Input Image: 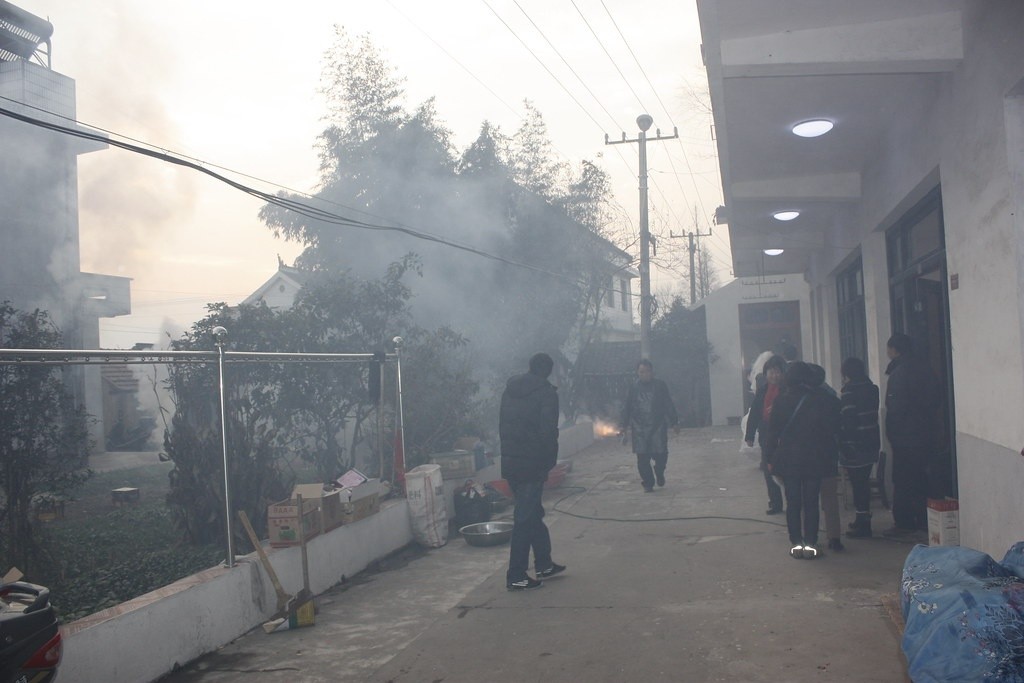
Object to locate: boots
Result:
[845,511,873,538]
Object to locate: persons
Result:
[882,333,929,536]
[839,358,880,540]
[738,342,841,561]
[618,359,680,492]
[499,352,566,592]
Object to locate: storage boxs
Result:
[35,500,63,523]
[431,450,473,478]
[455,437,486,471]
[113,487,139,509]
[295,483,348,534]
[339,470,380,525]
[268,496,316,549]
[927,496,959,547]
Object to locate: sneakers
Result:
[535,562,567,580]
[790,543,822,558]
[882,524,928,542]
[507,568,543,591]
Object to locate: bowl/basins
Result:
[458,522,513,546]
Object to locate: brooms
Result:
[236,505,309,624]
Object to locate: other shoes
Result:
[642,478,655,493]
[653,466,665,486]
[826,537,843,551]
[766,498,783,515]
